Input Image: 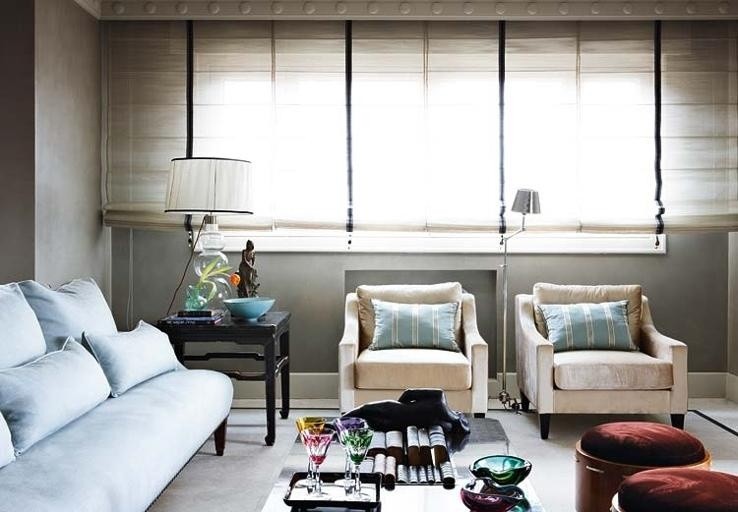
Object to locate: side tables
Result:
[158,312,290,445]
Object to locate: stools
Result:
[572,421,711,512]
[609,467,738,512]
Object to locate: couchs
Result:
[1,277,234,512]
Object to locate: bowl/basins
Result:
[460,477,525,512]
[468,455,532,487]
[223,297,276,322]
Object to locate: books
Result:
[158,309,222,324]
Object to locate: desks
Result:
[261,416,545,512]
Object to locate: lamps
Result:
[499,189,541,409]
[164,157,253,318]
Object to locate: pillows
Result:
[20,279,119,352]
[368,298,461,351]
[0,336,112,456]
[540,300,638,352]
[1,283,47,368]
[82,318,176,398]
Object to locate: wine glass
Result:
[295,416,327,485]
[340,427,373,501]
[332,417,368,486]
[300,428,336,500]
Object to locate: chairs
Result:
[515,283,688,440]
[339,279,489,418]
